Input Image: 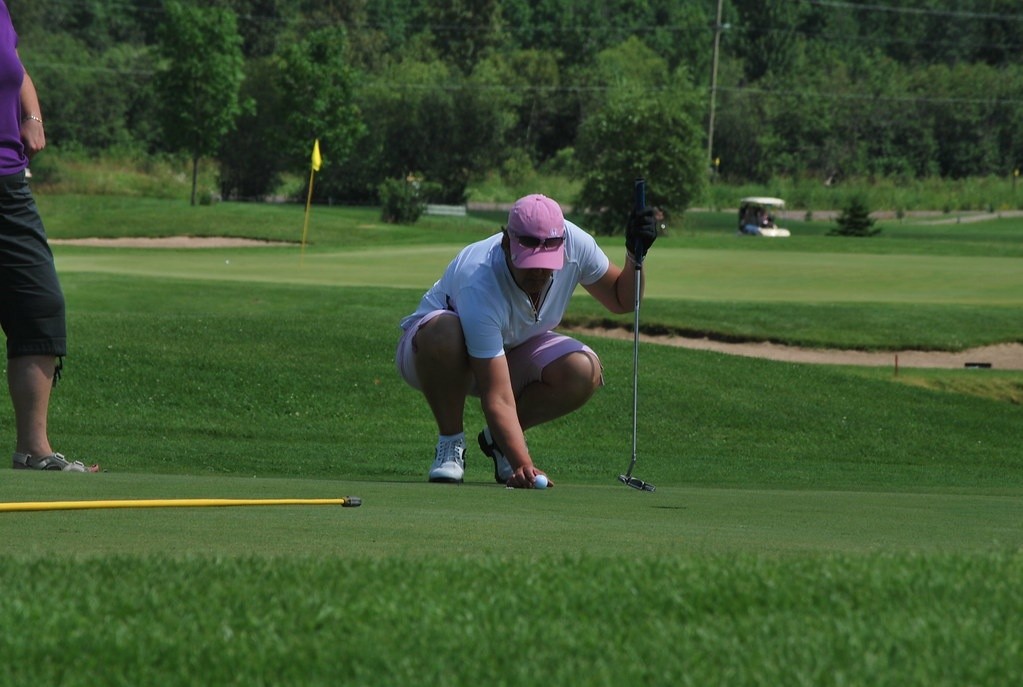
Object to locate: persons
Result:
[0,0,100,473]
[396,193,660,489]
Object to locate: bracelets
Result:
[23,116,42,123]
[626,252,645,263]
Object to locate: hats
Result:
[507,194,565,270]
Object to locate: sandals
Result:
[13,451,98,474]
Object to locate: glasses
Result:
[508,228,566,252]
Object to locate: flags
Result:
[311,139,322,171]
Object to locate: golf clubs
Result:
[0,495,363,513]
[617,176,657,492]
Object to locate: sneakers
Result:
[427,432,467,484]
[478,427,515,484]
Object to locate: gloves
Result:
[626,205,658,257]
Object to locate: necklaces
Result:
[526,290,541,317]
